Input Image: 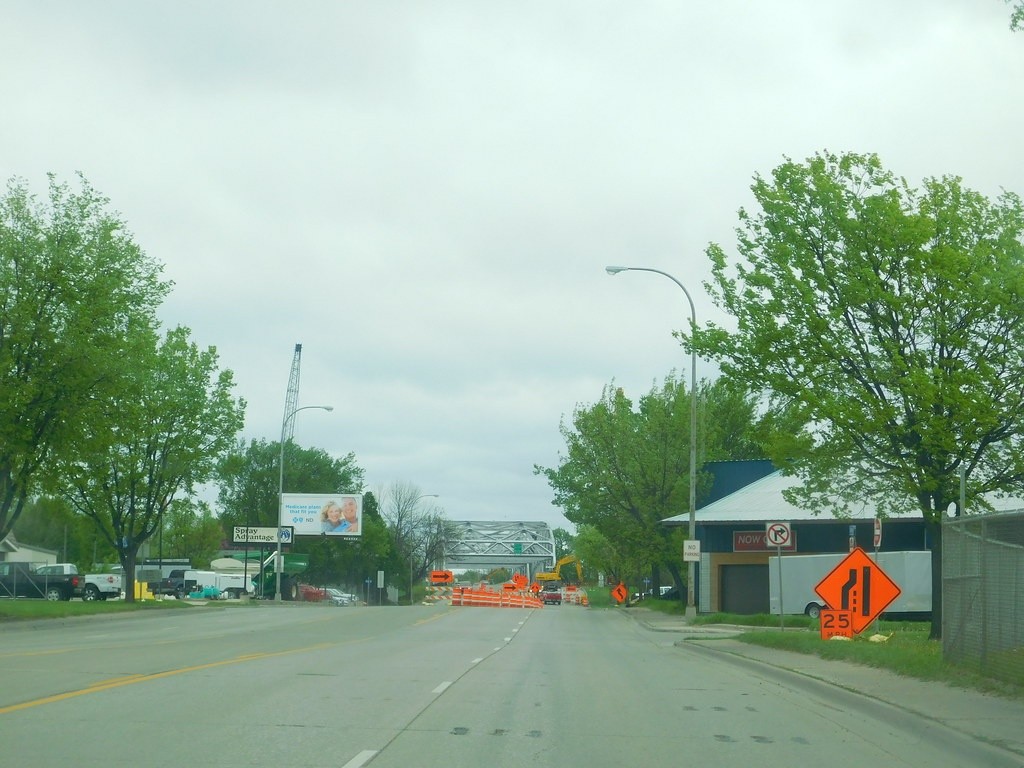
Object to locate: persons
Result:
[320,497,358,532]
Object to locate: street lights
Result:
[275,406,334,603]
[644,577,650,592]
[606,265,697,626]
[410,495,439,605]
[365,576,372,604]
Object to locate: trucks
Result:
[184,571,251,599]
[769,551,932,619]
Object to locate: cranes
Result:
[280,340,304,443]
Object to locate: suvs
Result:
[644,586,672,597]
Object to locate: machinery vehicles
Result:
[536,557,583,604]
[232,552,309,601]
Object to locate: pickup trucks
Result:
[33,564,121,602]
[146,569,196,600]
[0,562,78,602]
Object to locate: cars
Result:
[301,585,359,606]
